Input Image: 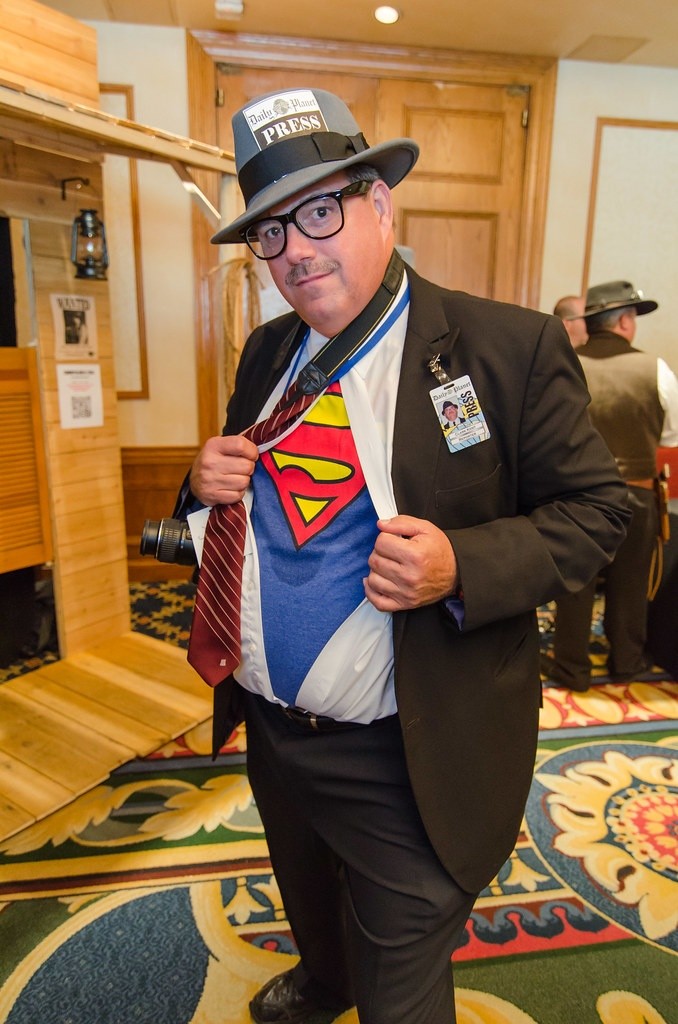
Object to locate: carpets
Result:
[0,579,678,1024]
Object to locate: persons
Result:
[173,88,631,1024]
[69,312,87,344]
[540,282,677,693]
[441,401,466,429]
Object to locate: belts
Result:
[244,691,402,736]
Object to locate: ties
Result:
[187,380,319,687]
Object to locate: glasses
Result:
[239,180,376,261]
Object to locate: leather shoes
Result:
[250,969,319,1023]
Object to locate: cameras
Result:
[140,517,200,584]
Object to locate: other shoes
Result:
[539,654,587,690]
[613,654,653,684]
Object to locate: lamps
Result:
[61,177,110,281]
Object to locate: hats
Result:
[564,282,658,321]
[210,86,419,245]
